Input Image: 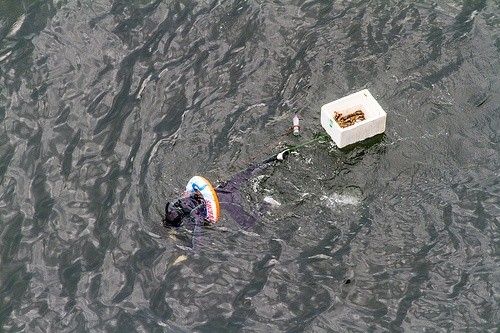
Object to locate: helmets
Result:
[166,210,182,227]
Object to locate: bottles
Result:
[292,113,300,136]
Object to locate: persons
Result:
[160,148,291,233]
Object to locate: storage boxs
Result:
[321,88,387,148]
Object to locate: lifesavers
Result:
[184,175,220,223]
